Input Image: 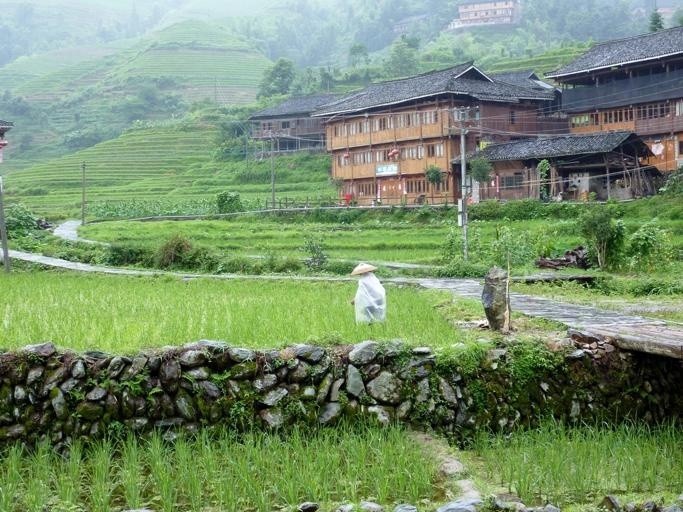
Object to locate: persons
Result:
[350,262,388,327]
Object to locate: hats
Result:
[350,262,377,275]
